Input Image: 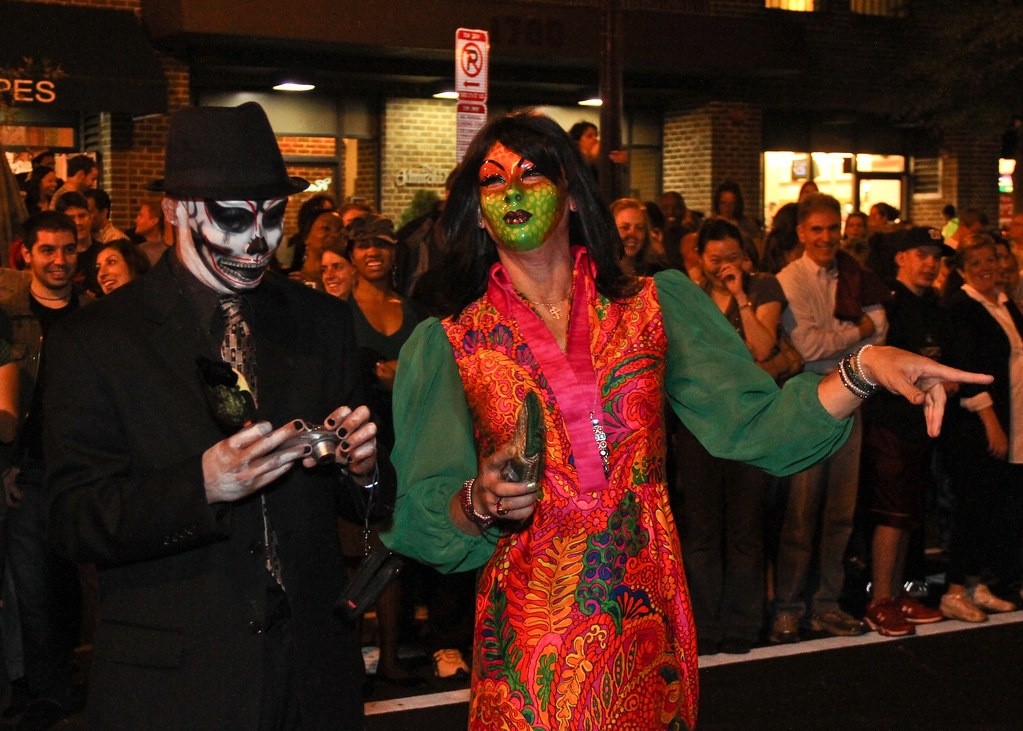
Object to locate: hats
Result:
[897,226,956,258]
[144,101,310,200]
[347,219,396,243]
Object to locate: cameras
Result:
[278,422,341,466]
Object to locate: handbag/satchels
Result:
[754,324,806,383]
[498,389,543,525]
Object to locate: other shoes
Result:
[375,668,422,687]
[697,637,752,655]
[14,700,64,731]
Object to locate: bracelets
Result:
[459,479,515,539]
[837,344,879,401]
[363,479,377,488]
[738,300,753,311]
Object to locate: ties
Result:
[216,295,287,594]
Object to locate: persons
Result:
[391,116,995,731]
[0,123,459,675]
[43,100,392,731]
[769,192,893,639]
[936,233,1023,624]
[861,226,957,638]
[567,121,1022,295]
[671,218,788,655]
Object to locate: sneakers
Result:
[939,594,987,622]
[862,599,916,636]
[810,608,863,635]
[433,648,469,680]
[770,613,800,643]
[973,585,1015,613]
[894,591,943,624]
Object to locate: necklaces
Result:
[29,283,73,300]
[515,285,572,319]
[585,378,609,472]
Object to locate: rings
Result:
[496,497,510,515]
